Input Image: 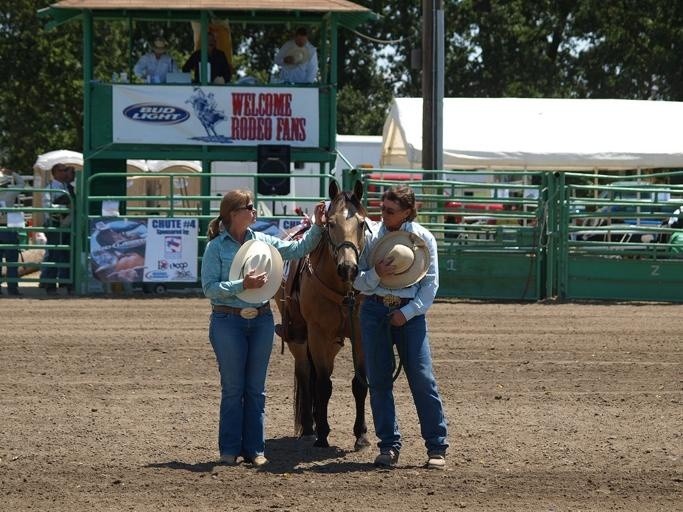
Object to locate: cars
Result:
[0,174,35,209]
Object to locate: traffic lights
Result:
[254,144,291,196]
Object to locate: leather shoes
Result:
[67,286,73,295]
[7,288,22,295]
[46,288,59,295]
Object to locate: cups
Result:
[119,73,127,83]
[170,59,177,73]
[111,73,118,83]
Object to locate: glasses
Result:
[235,205,253,210]
[380,205,394,214]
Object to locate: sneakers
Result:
[250,455,269,467]
[219,454,235,465]
[374,447,398,466]
[427,449,445,470]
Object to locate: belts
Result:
[212,303,269,320]
[358,294,413,310]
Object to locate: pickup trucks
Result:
[564,182,683,213]
[362,174,518,240]
[568,180,682,243]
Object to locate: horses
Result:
[275,176,372,456]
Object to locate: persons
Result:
[201,189,327,468]
[183,31,231,83]
[275,26,318,83]
[132,37,179,84]
[352,185,449,470]
[0,168,25,295]
[39,163,74,292]
[64,166,76,198]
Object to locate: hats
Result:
[368,230,430,290]
[149,35,169,53]
[227,239,283,303]
[286,47,306,68]
